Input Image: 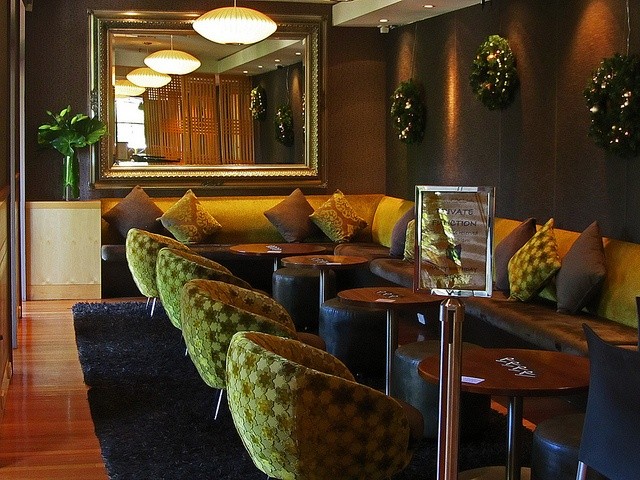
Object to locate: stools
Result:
[318,297,388,391]
[391,339,483,438]
[531,415,605,479]
[272,268,336,338]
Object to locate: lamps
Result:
[192,4,278,47]
[127,65,172,88]
[115,77,146,98]
[145,47,200,75]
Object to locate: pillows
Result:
[263,188,316,244]
[388,206,416,256]
[402,218,416,264]
[156,188,223,247]
[102,185,164,240]
[308,189,369,243]
[494,217,537,290]
[555,220,608,315]
[506,217,561,304]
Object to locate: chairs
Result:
[577,324,640,480]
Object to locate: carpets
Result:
[72,300,533,480]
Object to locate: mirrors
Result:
[415,184,494,297]
[85,8,329,192]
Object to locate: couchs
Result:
[333,195,640,364]
[227,330,413,479]
[126,229,200,317]
[99,195,384,300]
[181,280,326,421]
[154,247,269,355]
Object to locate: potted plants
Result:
[36,103,108,200]
